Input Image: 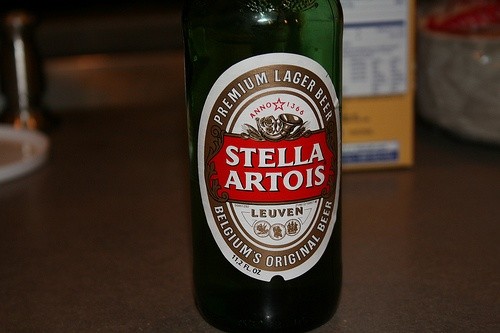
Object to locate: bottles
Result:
[182,0,344,333]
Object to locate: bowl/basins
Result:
[0,125,50,183]
